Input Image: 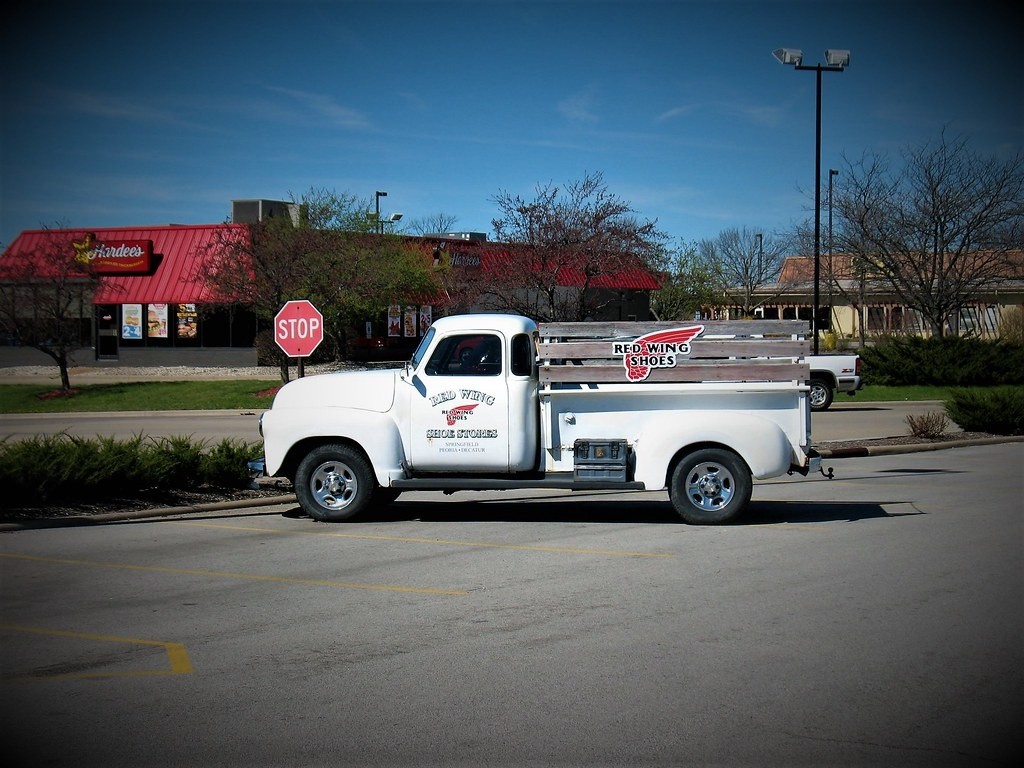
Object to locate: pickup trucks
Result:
[257,313,837,526]
[700,332,866,412]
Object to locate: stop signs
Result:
[275,299,325,357]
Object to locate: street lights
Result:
[771,47,854,355]
[374,188,388,241]
[756,233,763,274]
[827,169,841,334]
